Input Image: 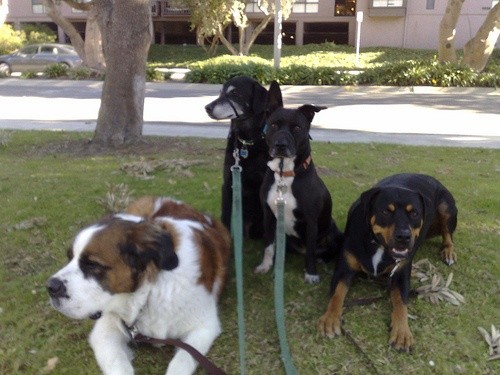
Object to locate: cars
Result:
[0,42,82,75]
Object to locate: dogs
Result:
[316,173,458,355]
[205,72,345,286]
[45,194,235,375]
